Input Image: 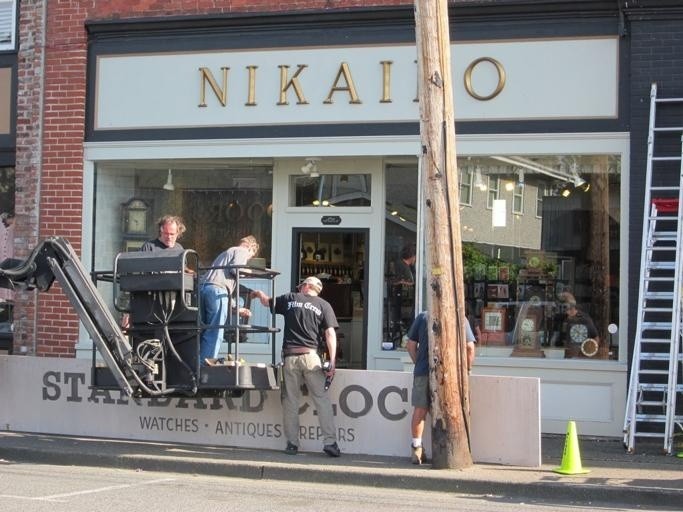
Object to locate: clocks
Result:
[564,320,588,358]
[120,197,153,236]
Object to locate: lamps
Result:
[474,156,586,188]
[163,168,175,191]
[302,159,321,179]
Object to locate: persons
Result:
[404,285,478,468]
[547,291,599,350]
[139,212,188,293]
[387,243,416,328]
[246,274,343,457]
[195,233,259,366]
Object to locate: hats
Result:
[296,277,323,291]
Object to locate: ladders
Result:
[622,84,683,456]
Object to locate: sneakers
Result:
[411,443,426,464]
[324,442,340,456]
[286,444,297,454]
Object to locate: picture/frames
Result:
[481,308,506,332]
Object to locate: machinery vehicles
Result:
[2,234,279,398]
